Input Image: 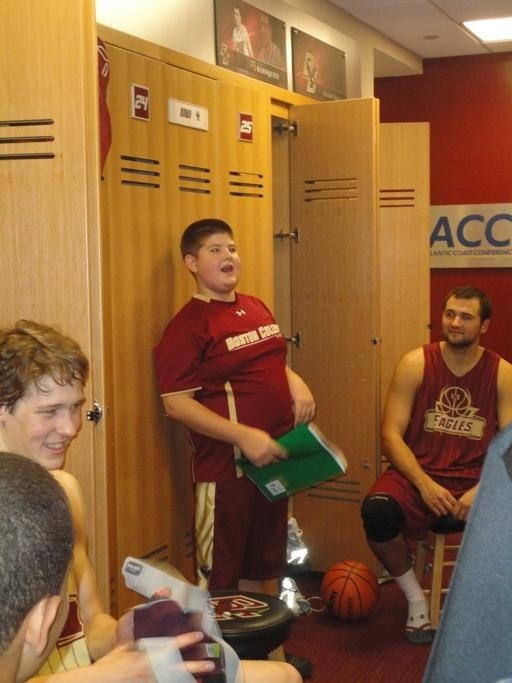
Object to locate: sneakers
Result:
[283,651,312,677]
[404,598,433,645]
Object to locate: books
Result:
[235,421,348,502]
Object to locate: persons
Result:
[154,219,318,674]
[360,287,512,647]
[256,14,285,69]
[0,451,75,683]
[0,318,303,683]
[233,6,255,73]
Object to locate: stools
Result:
[411,519,467,630]
[204,589,293,660]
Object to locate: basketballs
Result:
[322,561,377,619]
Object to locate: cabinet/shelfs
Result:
[0,0,431,622]
